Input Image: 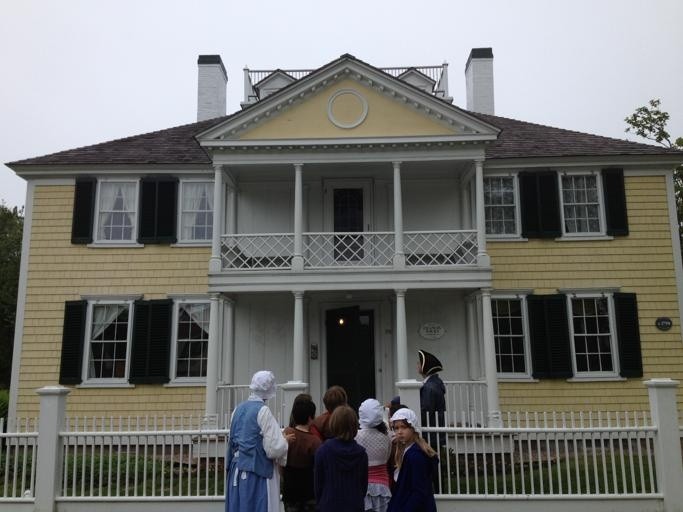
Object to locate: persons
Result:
[224,370,298,512]
[277,384,439,512]
[416,350,447,495]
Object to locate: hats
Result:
[418,350,442,376]
[359,399,383,429]
[391,408,422,439]
[250,370,277,400]
